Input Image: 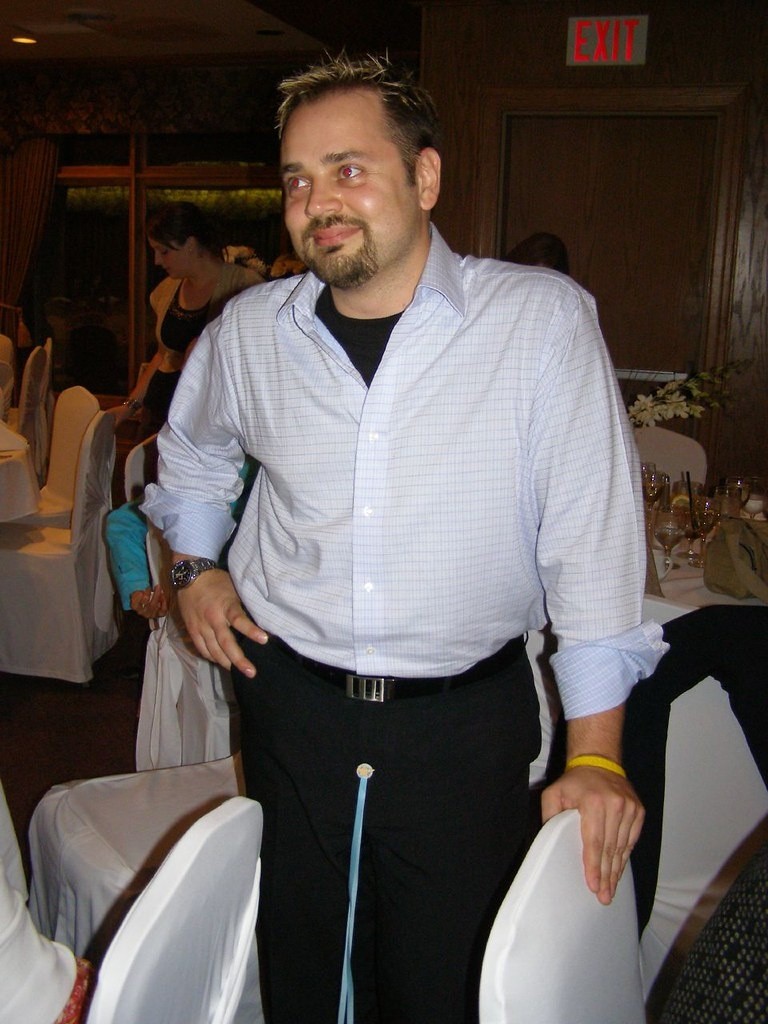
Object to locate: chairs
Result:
[134,527,242,775]
[12,384,99,529]
[633,426,709,502]
[635,591,768,1001]
[0,335,15,421]
[36,336,57,439]
[0,410,119,687]
[0,344,47,477]
[81,797,267,1024]
[27,748,250,972]
[479,807,649,1024]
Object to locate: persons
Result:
[136,57,671,1024]
[501,232,569,276]
[105,461,250,619]
[104,197,267,437]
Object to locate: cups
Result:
[648,544,673,584]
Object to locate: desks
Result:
[0,419,43,524]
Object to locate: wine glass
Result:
[639,460,768,568]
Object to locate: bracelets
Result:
[565,756,627,780]
[122,399,141,412]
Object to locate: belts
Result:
[267,627,524,697]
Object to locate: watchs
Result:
[168,558,219,592]
